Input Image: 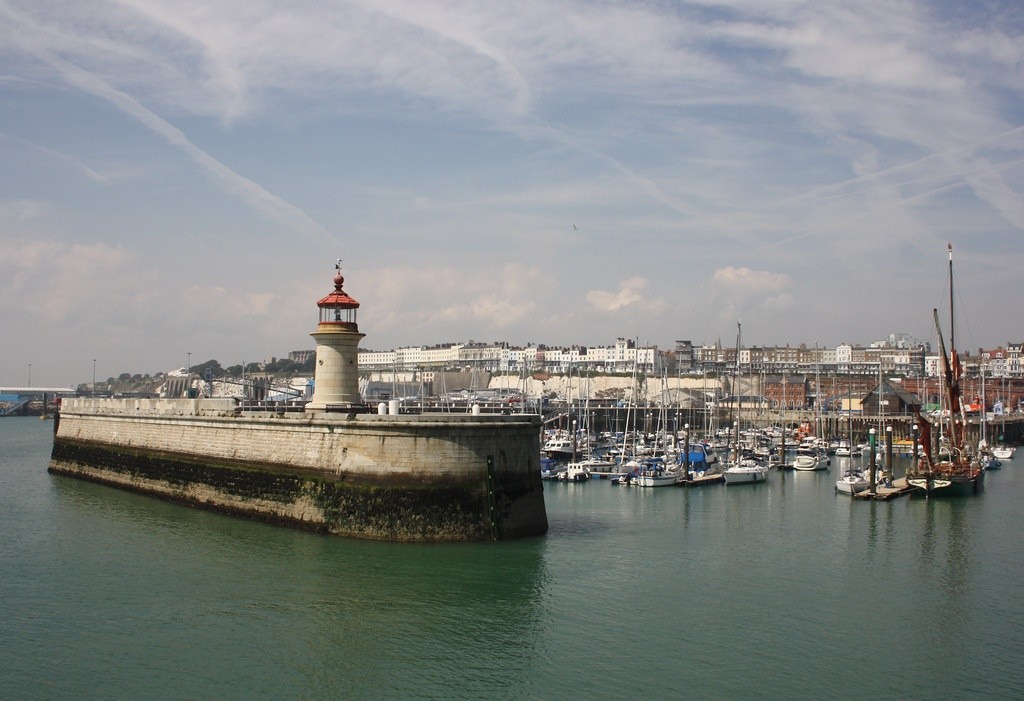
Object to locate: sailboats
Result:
[394,240,1017,499]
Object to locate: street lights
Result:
[28,363,32,387]
[93,359,97,397]
[186,352,192,378]
